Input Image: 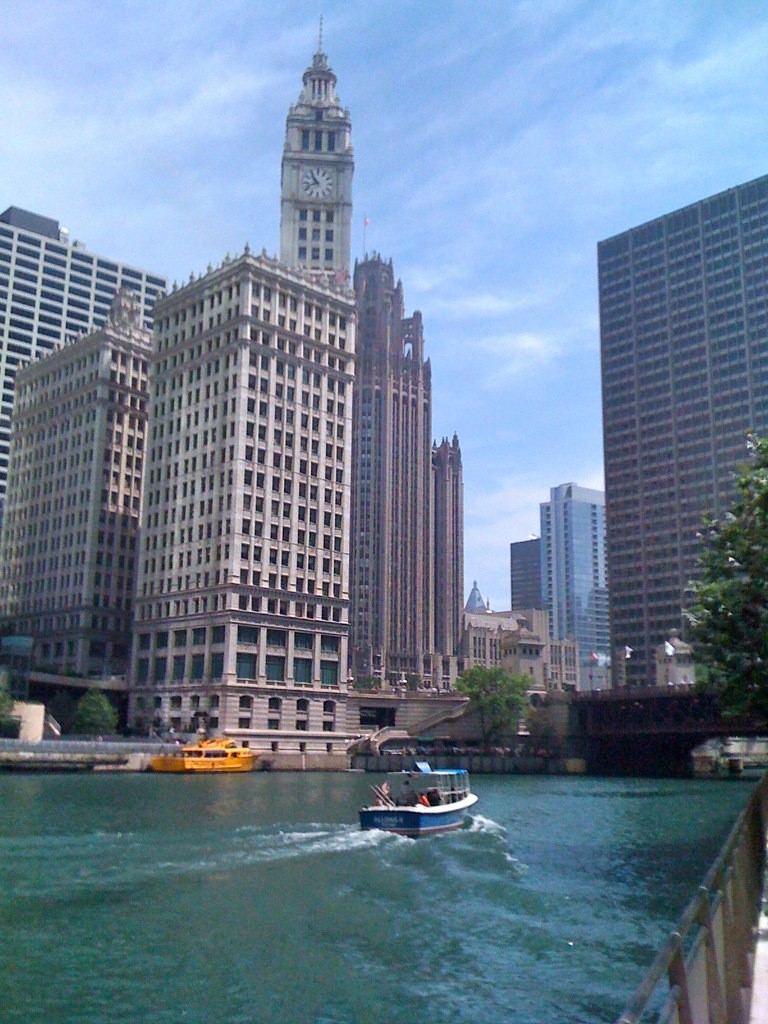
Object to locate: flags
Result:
[365,217,372,224]
[665,641,675,656]
[625,646,633,658]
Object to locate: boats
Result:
[358,761,480,839]
[148,739,262,773]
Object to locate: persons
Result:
[395,781,418,807]
[418,787,458,807]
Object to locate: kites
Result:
[591,652,598,661]
